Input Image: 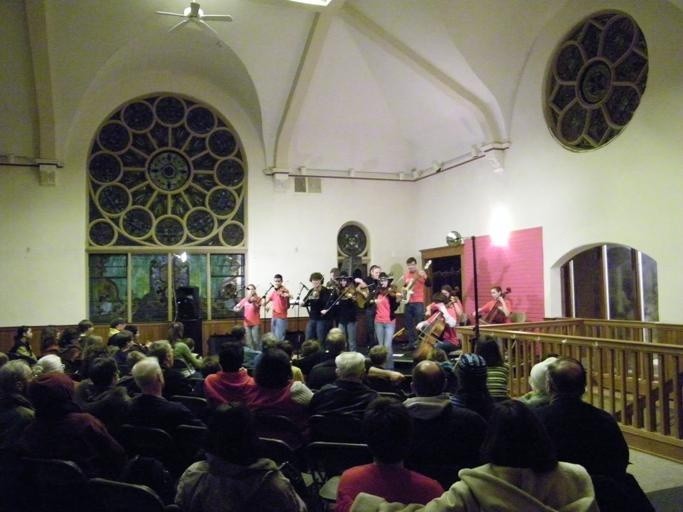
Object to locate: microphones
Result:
[241,285,249,290]
[270,282,277,291]
[300,282,309,291]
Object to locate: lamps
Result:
[172,251,189,263]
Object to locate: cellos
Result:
[478,288,512,345]
[414,299,455,362]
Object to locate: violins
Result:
[379,285,407,300]
[308,286,320,301]
[342,287,357,299]
[278,286,292,298]
[248,294,262,306]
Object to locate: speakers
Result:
[176,286,199,321]
[179,321,202,359]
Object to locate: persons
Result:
[350,397,599,511]
[516,357,561,406]
[427,334,512,415]
[1,320,446,512]
[234,259,512,370]
[530,356,653,511]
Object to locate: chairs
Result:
[174,423,214,454]
[18,456,78,508]
[119,365,129,372]
[121,424,169,455]
[378,391,403,400]
[172,396,206,413]
[191,377,204,391]
[304,440,370,466]
[257,436,290,461]
[80,476,166,512]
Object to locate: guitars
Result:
[395,261,432,303]
[345,272,392,308]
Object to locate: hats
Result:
[374,273,393,283]
[335,271,352,279]
[456,353,487,387]
[28,374,75,408]
